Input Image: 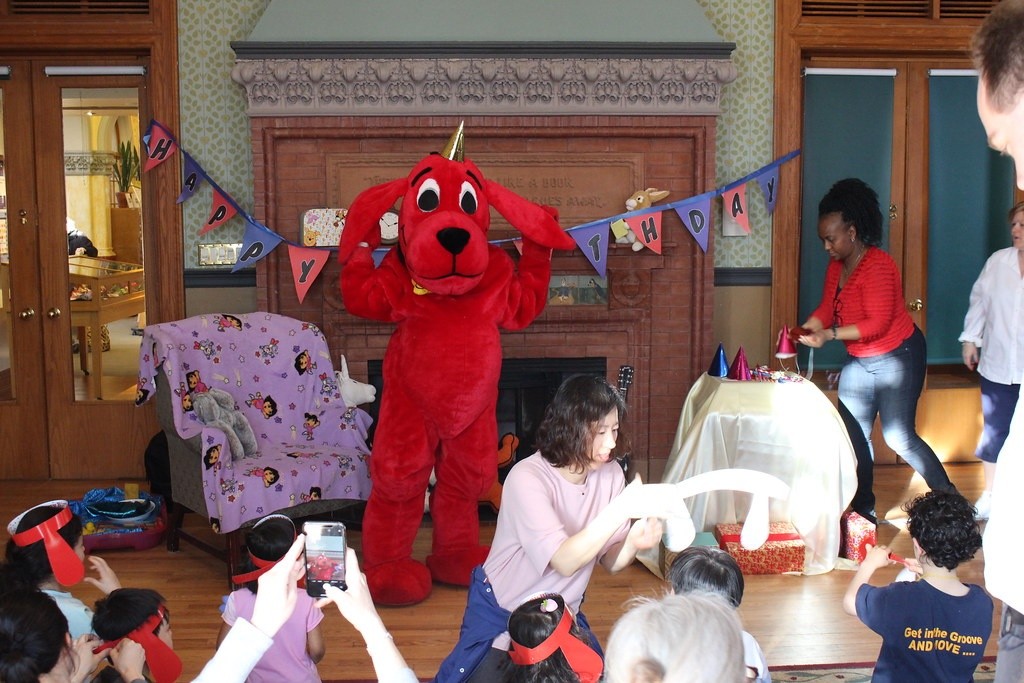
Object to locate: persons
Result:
[948,0,1024,682]
[92,587,176,683]
[0,572,149,683]
[603,587,747,683]
[185,527,426,683]
[210,512,328,683]
[773,176,964,530]
[505,590,599,683]
[440,366,669,682]
[841,488,996,682]
[954,198,1024,524]
[4,497,125,638]
[668,544,773,683]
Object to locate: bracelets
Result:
[367,629,394,655]
[830,326,838,341]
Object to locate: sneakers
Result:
[972,491,993,521]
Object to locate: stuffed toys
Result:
[191,383,260,461]
[307,551,339,582]
[329,116,578,608]
[611,184,671,252]
[336,351,378,406]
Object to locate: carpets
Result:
[321,655,997,682]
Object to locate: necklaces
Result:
[840,242,869,279]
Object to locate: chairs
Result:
[134,312,370,590]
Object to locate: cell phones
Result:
[303,521,347,598]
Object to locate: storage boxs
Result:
[68,498,168,554]
[842,511,876,562]
[660,532,719,580]
[714,521,805,575]
